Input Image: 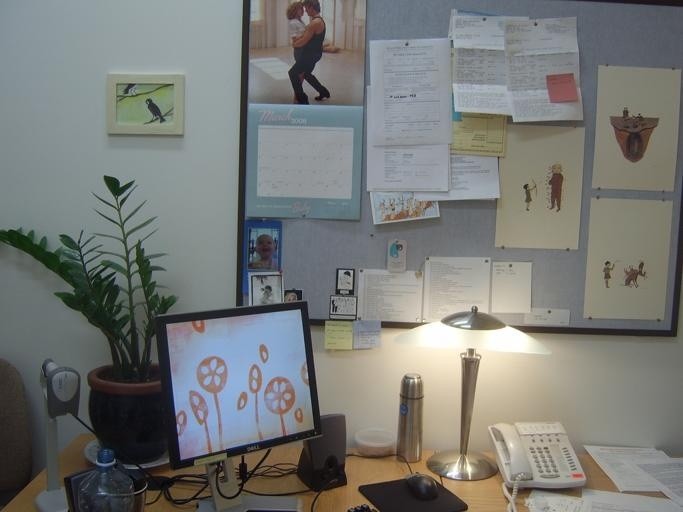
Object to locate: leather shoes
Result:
[315,87,330,100]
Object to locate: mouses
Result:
[407,473,439,500]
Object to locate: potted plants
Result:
[1,175,178,464]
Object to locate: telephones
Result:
[488,421,587,488]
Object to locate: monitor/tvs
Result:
[153,301,322,512]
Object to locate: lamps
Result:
[435,462,437,469]
[425,305,507,481]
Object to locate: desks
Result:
[1,435,668,512]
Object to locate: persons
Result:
[286,0,331,104]
[248,234,276,269]
[259,285,298,304]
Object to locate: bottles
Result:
[396,372,424,462]
[78,449,135,512]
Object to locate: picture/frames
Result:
[243,219,282,295]
[105,74,185,135]
[248,272,284,306]
[284,288,302,302]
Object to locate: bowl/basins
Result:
[354,428,396,457]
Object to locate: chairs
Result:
[1,358,32,511]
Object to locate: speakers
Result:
[298,413,347,492]
